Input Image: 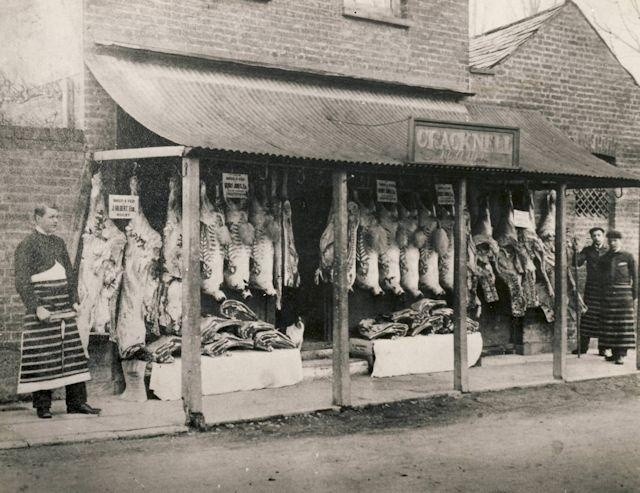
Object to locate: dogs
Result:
[280,313,307,351]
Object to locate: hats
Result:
[606,230,621,238]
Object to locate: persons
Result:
[10,201,102,417]
[571,226,608,356]
[594,230,640,364]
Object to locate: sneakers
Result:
[573,349,586,354]
[598,349,623,364]
[38,407,52,418]
[68,404,101,414]
